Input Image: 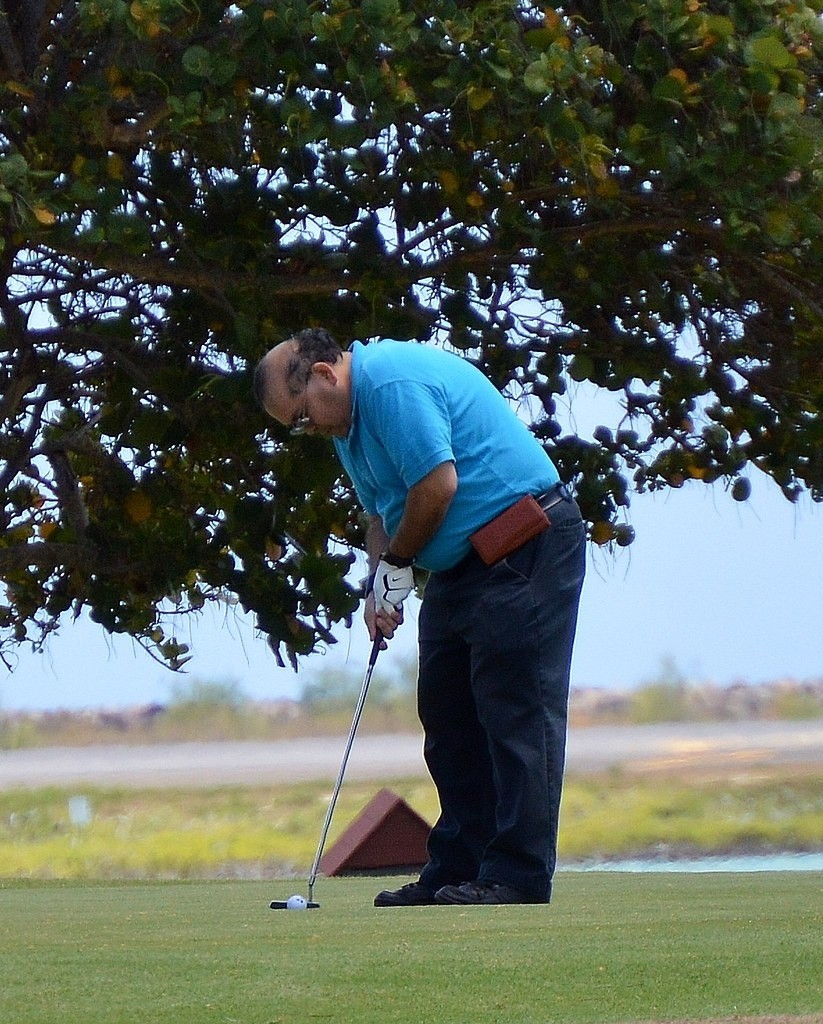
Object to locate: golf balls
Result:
[286,894,307,911]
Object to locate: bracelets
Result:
[364,573,375,597]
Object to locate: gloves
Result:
[373,551,414,615]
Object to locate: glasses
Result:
[290,371,312,437]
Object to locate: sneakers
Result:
[374,877,438,906]
[434,878,548,904]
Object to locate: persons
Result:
[254,324,588,905]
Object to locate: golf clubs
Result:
[270,627,383,909]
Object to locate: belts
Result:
[536,486,567,511]
